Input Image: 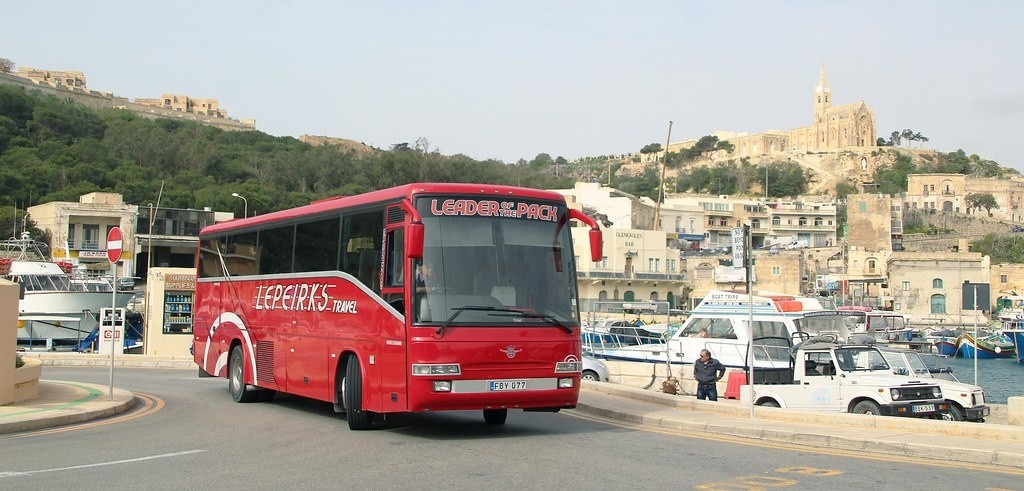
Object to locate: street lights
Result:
[231,192,248,219]
[765,158,769,198]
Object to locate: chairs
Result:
[805,360,822,376]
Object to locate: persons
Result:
[694,349,725,402]
[416,259,437,293]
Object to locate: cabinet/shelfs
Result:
[165,301,193,333]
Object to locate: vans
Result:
[785,240,805,249]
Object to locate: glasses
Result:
[700,354,708,357]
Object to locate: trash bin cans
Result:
[99,307,125,354]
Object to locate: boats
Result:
[934,335,957,356]
[1002,315,1024,365]
[955,332,1015,359]
[0,261,136,343]
[836,305,913,349]
[578,288,953,400]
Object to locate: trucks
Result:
[622,299,657,315]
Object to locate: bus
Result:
[191,182,603,431]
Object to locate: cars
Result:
[582,352,609,383]
[1013,227,1022,232]
[117,276,135,291]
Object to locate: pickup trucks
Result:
[739,344,951,419]
[856,346,991,423]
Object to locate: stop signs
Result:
[106,227,123,263]
